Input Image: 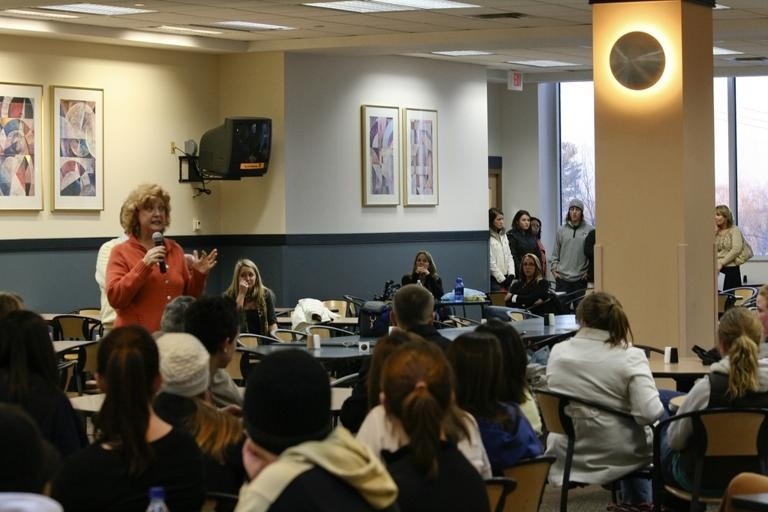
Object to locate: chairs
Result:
[506,310,542,320]
[343,294,367,318]
[494,455,557,512]
[223,348,264,387]
[485,477,517,512]
[654,409,768,512]
[270,329,308,343]
[448,315,483,327]
[320,298,350,317]
[532,386,657,512]
[52,315,105,393]
[57,360,79,395]
[305,324,356,337]
[720,286,759,308]
[718,293,737,319]
[237,333,279,347]
[328,372,361,427]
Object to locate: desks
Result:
[51,339,98,354]
[273,315,579,359]
[436,299,491,319]
[276,316,359,326]
[38,313,102,330]
[647,356,711,393]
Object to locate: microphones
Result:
[152,231,167,274]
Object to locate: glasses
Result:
[523,262,535,266]
[531,223,540,227]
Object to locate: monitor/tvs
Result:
[198,115,272,178]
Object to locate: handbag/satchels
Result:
[728,226,753,265]
[344,294,391,337]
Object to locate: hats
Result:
[568,199,583,211]
[244,349,334,454]
[156,333,210,397]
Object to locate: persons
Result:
[0,403,66,512]
[153,331,247,477]
[372,340,491,512]
[0,292,24,313]
[489,208,515,294]
[543,291,666,512]
[402,250,444,298]
[188,294,243,407]
[449,331,543,475]
[151,296,244,408]
[476,319,543,434]
[529,216,547,277]
[105,184,218,335]
[757,285,768,343]
[716,205,741,291]
[505,253,548,310]
[551,198,595,310]
[221,258,278,336]
[338,284,459,432]
[1,310,88,453]
[96,195,131,340]
[230,347,402,512]
[55,323,208,512]
[364,327,493,479]
[507,210,539,278]
[658,305,768,498]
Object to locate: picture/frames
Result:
[50,85,104,212]
[0,81,44,213]
[362,104,401,207]
[403,107,440,206]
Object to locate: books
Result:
[718,272,726,292]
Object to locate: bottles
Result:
[146,486,167,511]
[454,277,464,302]
